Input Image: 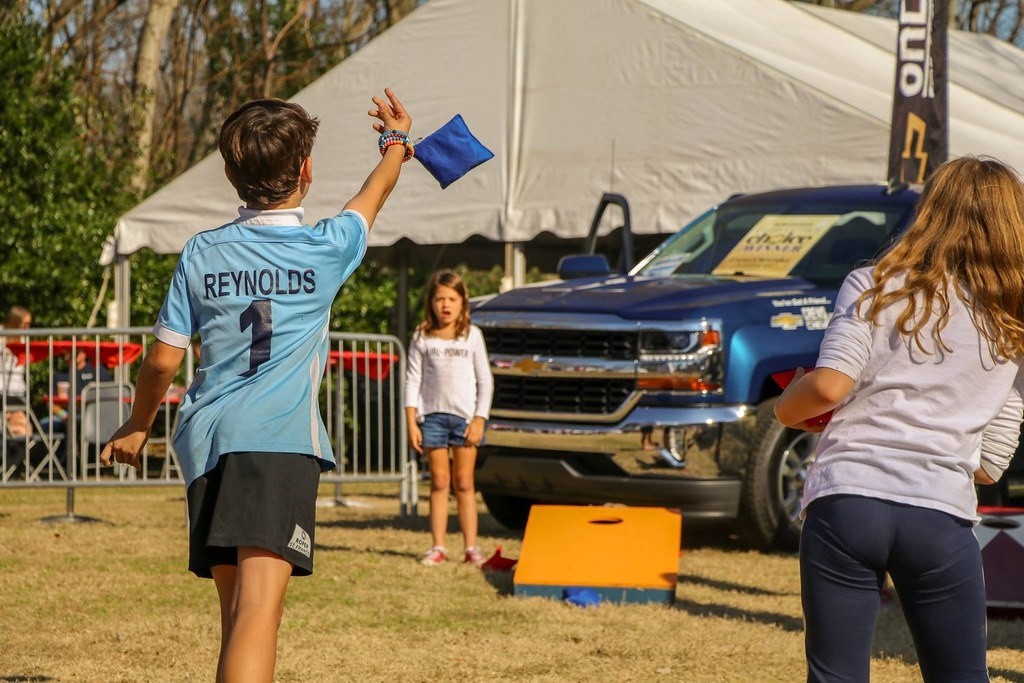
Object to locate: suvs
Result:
[466,186,924,551]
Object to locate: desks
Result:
[41,387,186,480]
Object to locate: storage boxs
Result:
[512,504,681,605]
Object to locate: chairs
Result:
[0,382,183,482]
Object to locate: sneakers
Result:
[464,546,486,568]
[421,546,449,565]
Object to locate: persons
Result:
[99,88,414,683]
[773,154,1024,682]
[404,268,495,567]
[35,351,113,470]
[0,305,32,481]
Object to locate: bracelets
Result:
[378,129,414,163]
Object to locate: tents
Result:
[98,0,1024,482]
